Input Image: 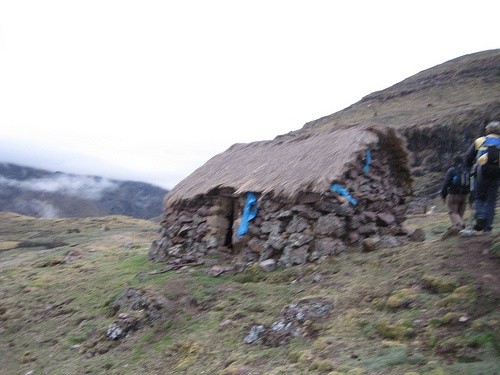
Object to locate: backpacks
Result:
[452,167,470,189]
[475,136,500,186]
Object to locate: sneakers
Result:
[473,217,483,230]
[484,225,491,232]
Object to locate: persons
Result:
[465,121,500,232]
[440,154,469,229]
